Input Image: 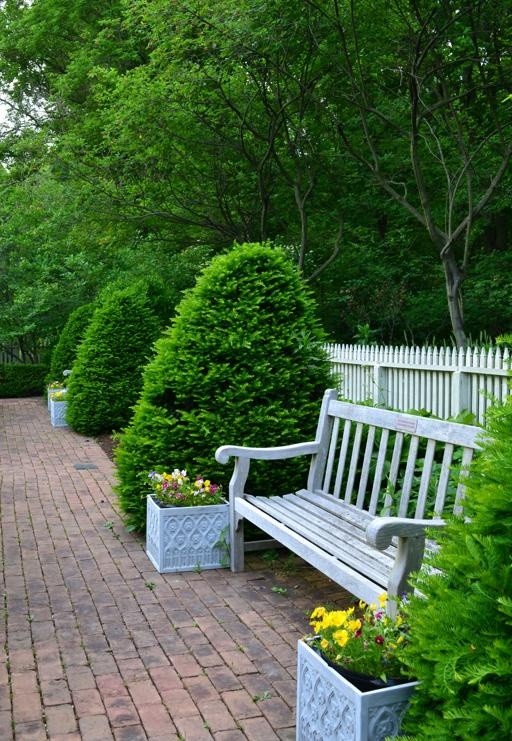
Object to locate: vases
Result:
[295,635,425,741]
[145,494,232,574]
[50,399,70,427]
[48,389,66,412]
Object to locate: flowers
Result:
[50,391,65,401]
[306,592,408,683]
[48,380,65,390]
[143,468,224,506]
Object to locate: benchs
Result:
[214,388,493,621]
